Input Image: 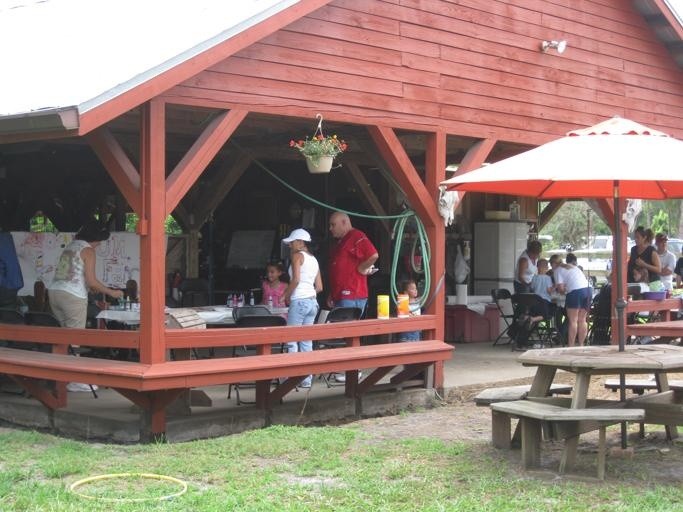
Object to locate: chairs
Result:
[0,308,25,394]
[233,306,299,392]
[314,307,363,389]
[23,312,98,398]
[228,316,287,405]
[492,288,518,344]
[511,293,552,352]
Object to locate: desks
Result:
[445,302,503,343]
[101,307,233,362]
[510,344,683,473]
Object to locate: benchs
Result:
[490,400,645,479]
[604,378,683,438]
[474,384,573,448]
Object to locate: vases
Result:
[306,155,336,174]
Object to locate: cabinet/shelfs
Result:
[473,222,527,338]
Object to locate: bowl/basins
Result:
[646,291,666,301]
[484,210,511,221]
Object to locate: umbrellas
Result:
[440,115,683,449]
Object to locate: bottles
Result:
[509,201,520,222]
[250,288,254,305]
[268,291,273,310]
[377,295,389,320]
[397,294,409,318]
[125,296,130,311]
[227,289,246,308]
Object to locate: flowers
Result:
[290,134,348,166]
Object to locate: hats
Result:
[76,222,111,242]
[655,233,668,244]
[282,228,312,244]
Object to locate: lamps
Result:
[540,39,566,53]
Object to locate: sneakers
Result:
[66,382,98,392]
[298,380,311,387]
[512,345,526,352]
[336,371,362,382]
[532,344,543,349]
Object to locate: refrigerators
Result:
[474,221,528,296]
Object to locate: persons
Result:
[627,267,650,292]
[514,240,543,318]
[654,233,676,291]
[282,228,323,388]
[566,253,577,265]
[524,259,552,325]
[0,230,24,307]
[262,262,288,308]
[546,254,568,331]
[399,279,425,387]
[674,246,683,288]
[628,226,665,292]
[549,255,592,347]
[48,227,124,392]
[326,212,379,381]
